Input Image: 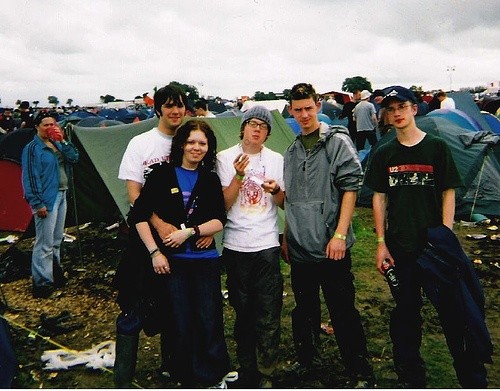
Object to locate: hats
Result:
[360,90,371,100]
[372,90,384,98]
[241,106,272,137]
[381,87,415,107]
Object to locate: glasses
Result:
[246,120,268,129]
[385,104,414,113]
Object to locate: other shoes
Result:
[48,290,62,298]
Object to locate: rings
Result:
[167,239,170,241]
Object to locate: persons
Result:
[282,83,376,388]
[339,83,500,153]
[22,112,79,298]
[194,101,216,118]
[365,87,494,389]
[117,86,213,273]
[127,121,238,388]
[216,106,285,387]
[0,101,64,137]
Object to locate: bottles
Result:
[381,258,397,285]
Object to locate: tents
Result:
[65,108,155,127]
[62,110,297,255]
[357,117,500,219]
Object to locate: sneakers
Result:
[280,361,312,377]
[351,379,370,389]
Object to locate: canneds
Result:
[381,258,402,289]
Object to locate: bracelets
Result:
[377,237,385,242]
[270,187,280,195]
[150,248,158,257]
[62,140,66,143]
[236,173,245,180]
[193,226,200,237]
[333,232,346,240]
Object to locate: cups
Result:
[45,126,61,141]
